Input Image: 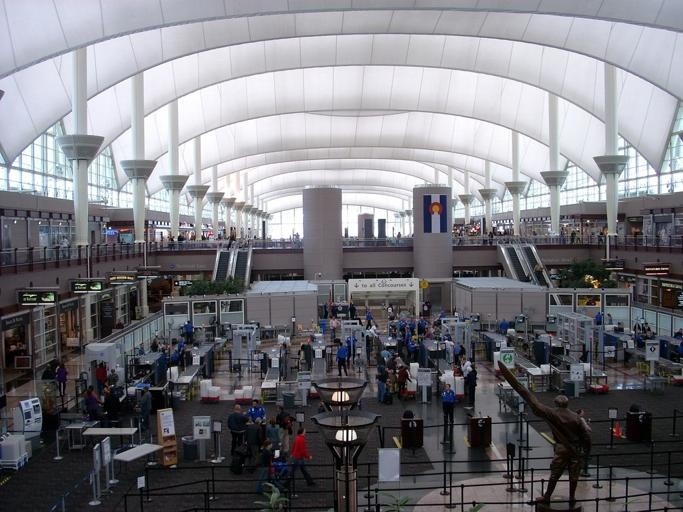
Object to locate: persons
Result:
[227,398,317,493]
[325,296,477,429]
[499,318,509,335]
[675,343,683,362]
[184,320,194,345]
[606,313,612,325]
[497,360,592,508]
[41,356,69,397]
[115,319,124,329]
[596,312,602,325]
[298,345,305,371]
[576,409,590,454]
[279,342,289,381]
[160,226,254,249]
[633,317,655,348]
[60,235,68,258]
[674,328,683,347]
[614,321,624,334]
[453,220,606,247]
[82,334,187,433]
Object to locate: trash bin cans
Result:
[282,391,296,408]
[181,435,198,460]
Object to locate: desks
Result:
[0,315,682,481]
